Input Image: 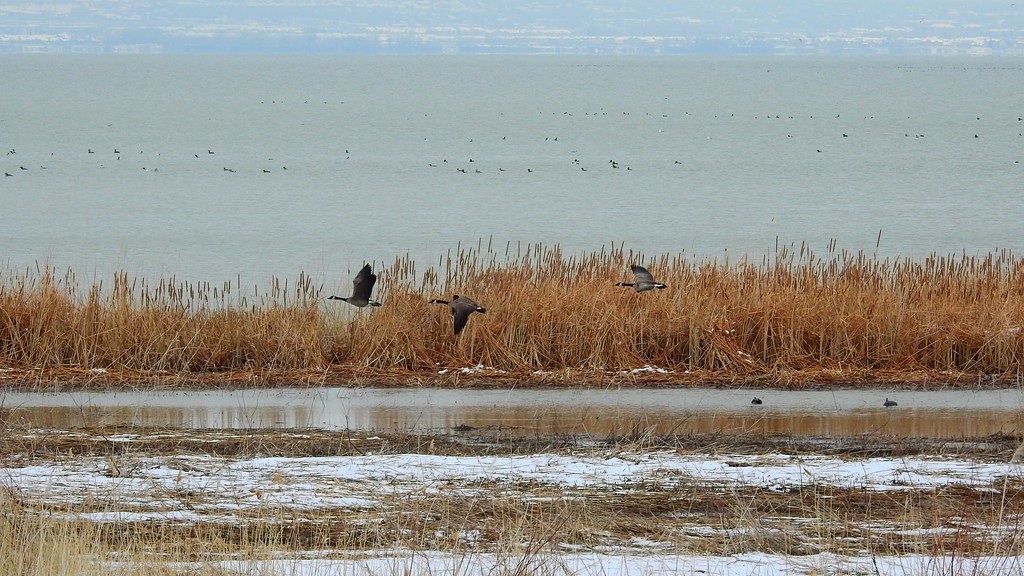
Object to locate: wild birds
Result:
[752,110,1023,164]
[427,294,487,337]
[327,262,383,308]
[751,396,762,405]
[882,397,898,407]
[614,264,669,295]
[422,107,736,175]
[0,100,350,178]
